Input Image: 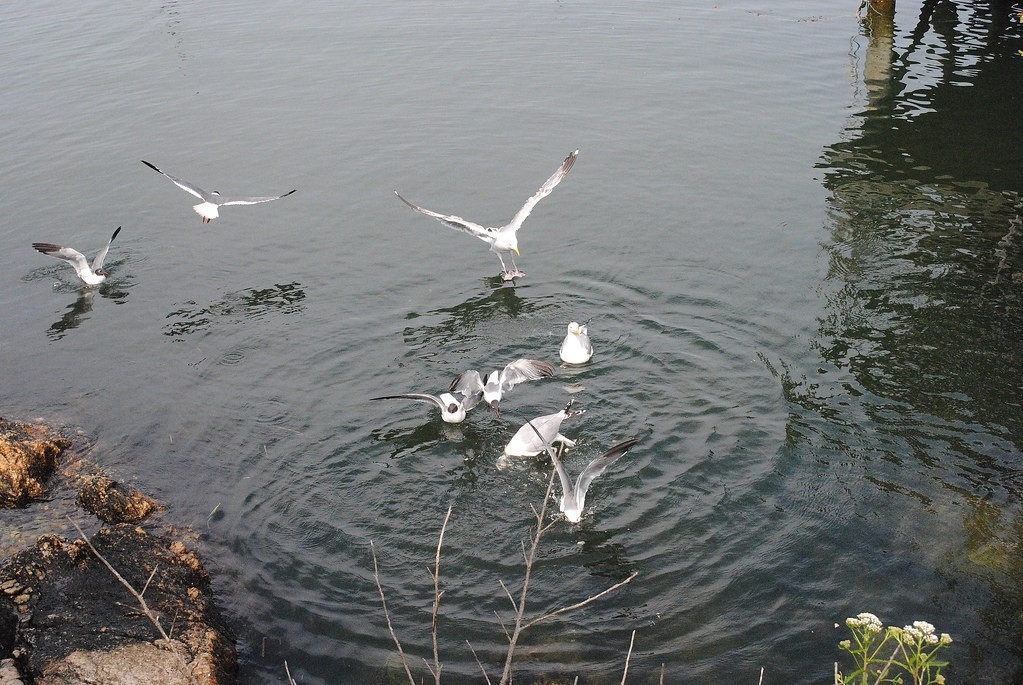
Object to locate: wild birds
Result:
[523,417,639,525]
[449,359,556,418]
[32,226,122,285]
[505,398,587,457]
[393,148,579,281]
[559,317,594,365]
[369,373,488,424]
[141,160,297,224]
